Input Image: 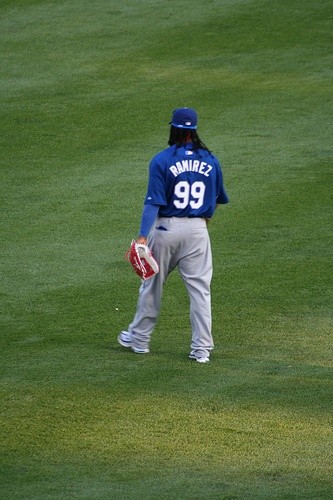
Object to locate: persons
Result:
[117,109,229,363]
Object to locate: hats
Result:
[168,108,197,129]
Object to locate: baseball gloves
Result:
[129,239,159,281]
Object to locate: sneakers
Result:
[189,351,209,363]
[117,331,149,353]
